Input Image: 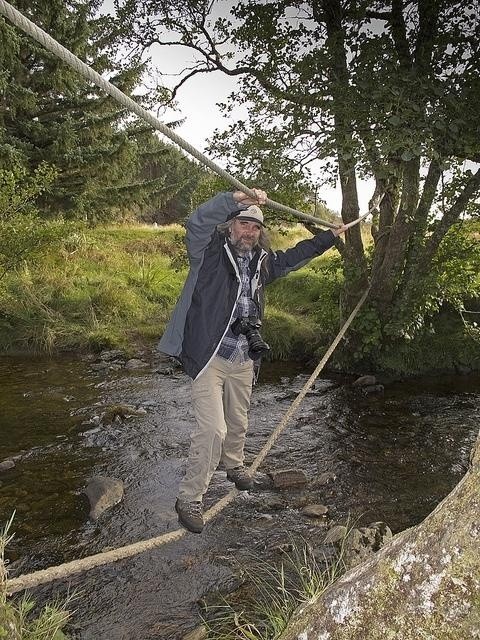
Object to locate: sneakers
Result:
[226,467,253,490]
[174,498,205,535]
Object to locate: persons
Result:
[153,186,348,534]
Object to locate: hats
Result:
[235,204,267,228]
